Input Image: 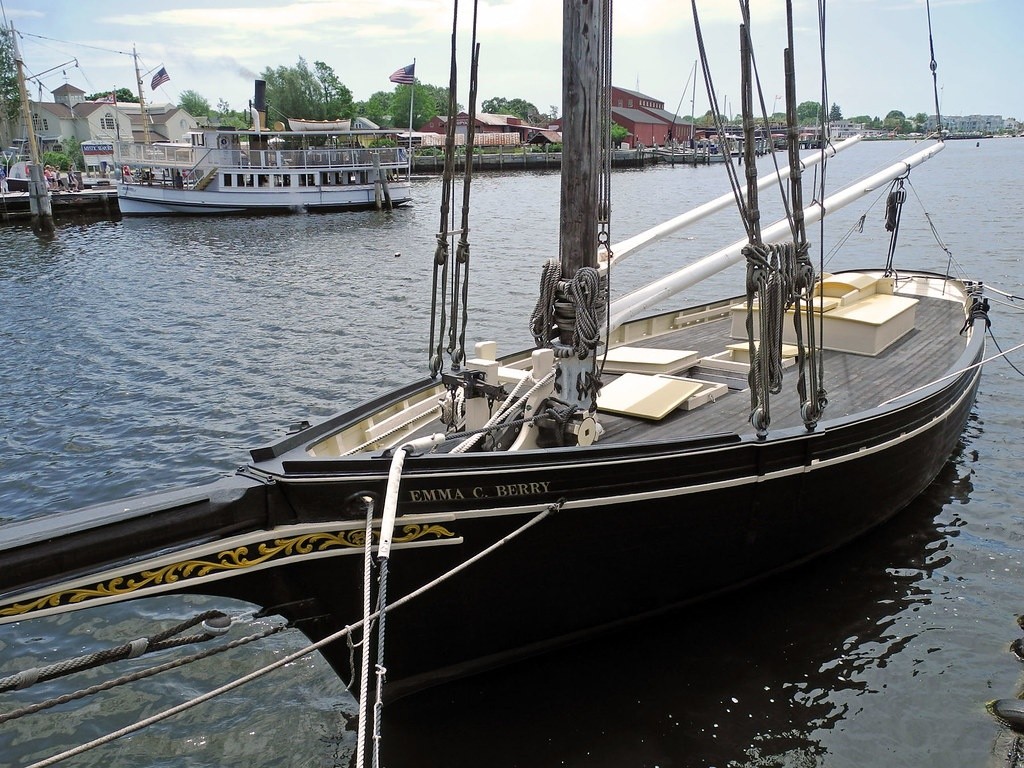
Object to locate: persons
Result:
[0,165,10,194]
[124,165,133,183]
[176,172,183,189]
[44,165,81,192]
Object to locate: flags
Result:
[389,64,414,84]
[151,68,170,91]
[93,94,116,104]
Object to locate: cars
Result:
[876,133,887,138]
[888,133,924,137]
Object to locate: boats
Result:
[117,79,414,214]
[933,129,953,140]
[4,177,117,189]
[287,117,353,132]
[1003,133,1024,137]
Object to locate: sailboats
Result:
[0,2,991,768]
[643,59,820,162]
